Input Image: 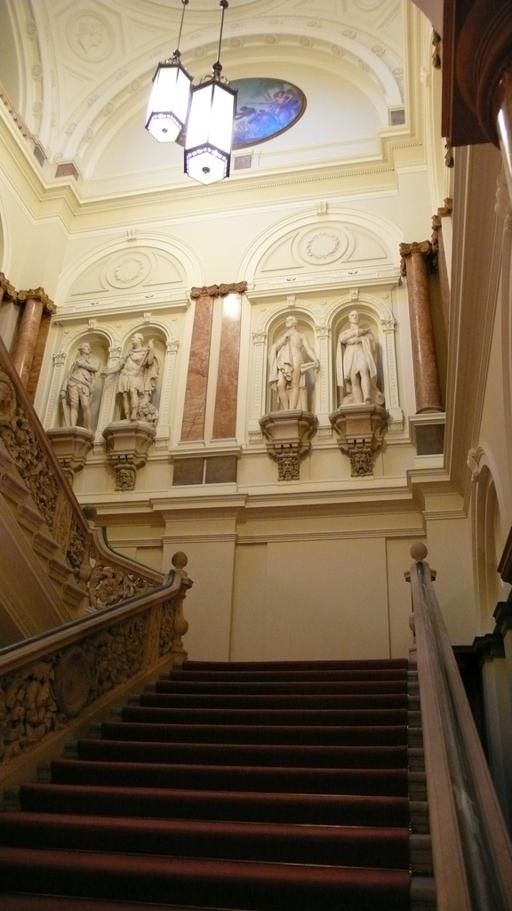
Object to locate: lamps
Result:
[141,1,196,149]
[179,0,241,190]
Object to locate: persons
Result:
[339,310,375,404]
[59,341,100,431]
[270,316,318,410]
[100,332,155,420]
[235,90,301,139]
